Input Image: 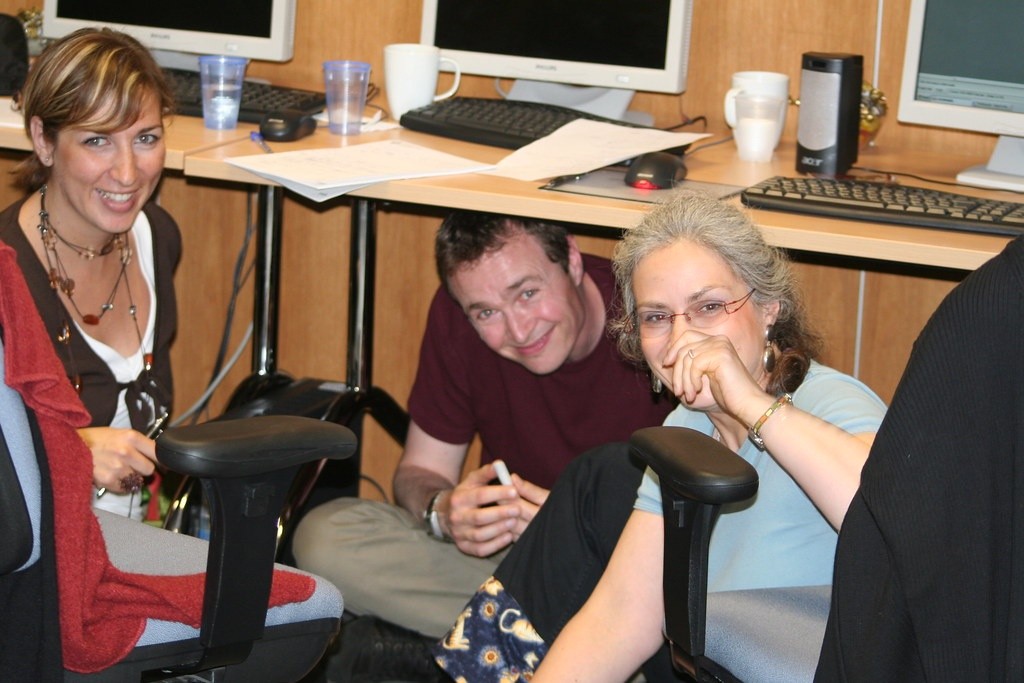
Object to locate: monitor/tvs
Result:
[420,0,693,129]
[43,0,299,73]
[896,0,1024,193]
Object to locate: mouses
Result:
[258,109,317,143]
[624,152,688,191]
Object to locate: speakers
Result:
[795,51,864,177]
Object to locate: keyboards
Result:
[400,94,691,167]
[739,175,1024,236]
[158,68,327,125]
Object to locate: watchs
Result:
[422,488,451,543]
[747,393,793,451]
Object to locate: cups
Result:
[724,70,790,163]
[324,61,371,135]
[197,56,247,129]
[383,44,460,122]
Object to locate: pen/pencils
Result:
[546,173,585,189]
[492,460,511,487]
[806,172,898,183]
[96,411,172,500]
[250,130,274,154]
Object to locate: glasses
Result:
[623,288,757,339]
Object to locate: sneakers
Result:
[324,616,456,683]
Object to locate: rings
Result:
[688,350,694,359]
[120,471,143,495]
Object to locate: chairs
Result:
[0,241,360,683]
[629,427,832,683]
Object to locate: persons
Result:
[291,211,682,683]
[435,181,889,683]
[813,232,1024,683]
[0,27,183,522]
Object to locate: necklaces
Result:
[38,183,153,396]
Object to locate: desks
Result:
[0,96,376,533]
[184,118,1024,566]
